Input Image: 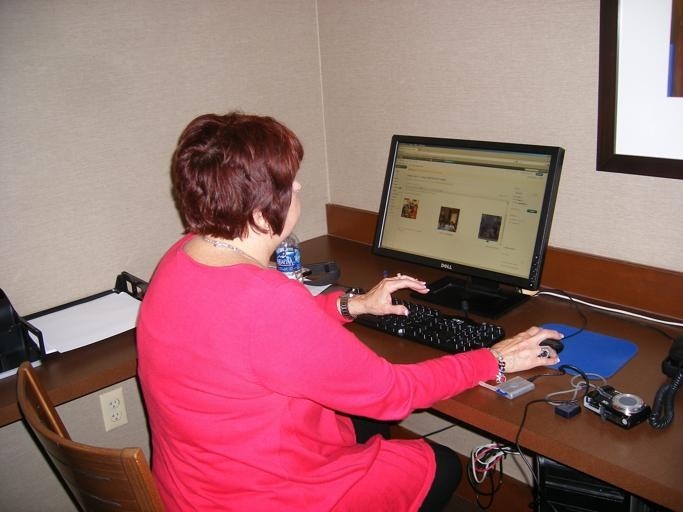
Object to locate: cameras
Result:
[585,382,652,430]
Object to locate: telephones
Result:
[662,332,683,380]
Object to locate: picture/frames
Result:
[595,0,683,179]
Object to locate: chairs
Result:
[16,360,167,512]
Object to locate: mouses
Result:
[540,338,565,356]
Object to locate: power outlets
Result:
[98,386,130,431]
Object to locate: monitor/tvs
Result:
[370,133,565,321]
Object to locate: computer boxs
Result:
[532,455,648,511]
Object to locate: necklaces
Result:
[198,234,266,269]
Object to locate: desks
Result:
[270,234,683,512]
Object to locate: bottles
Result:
[275,232,304,285]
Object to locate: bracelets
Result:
[339,286,365,322]
[485,346,507,383]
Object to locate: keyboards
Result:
[353,294,505,357]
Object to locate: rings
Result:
[537,344,551,359]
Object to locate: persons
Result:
[408,199,417,219]
[436,212,456,231]
[133,109,567,511]
